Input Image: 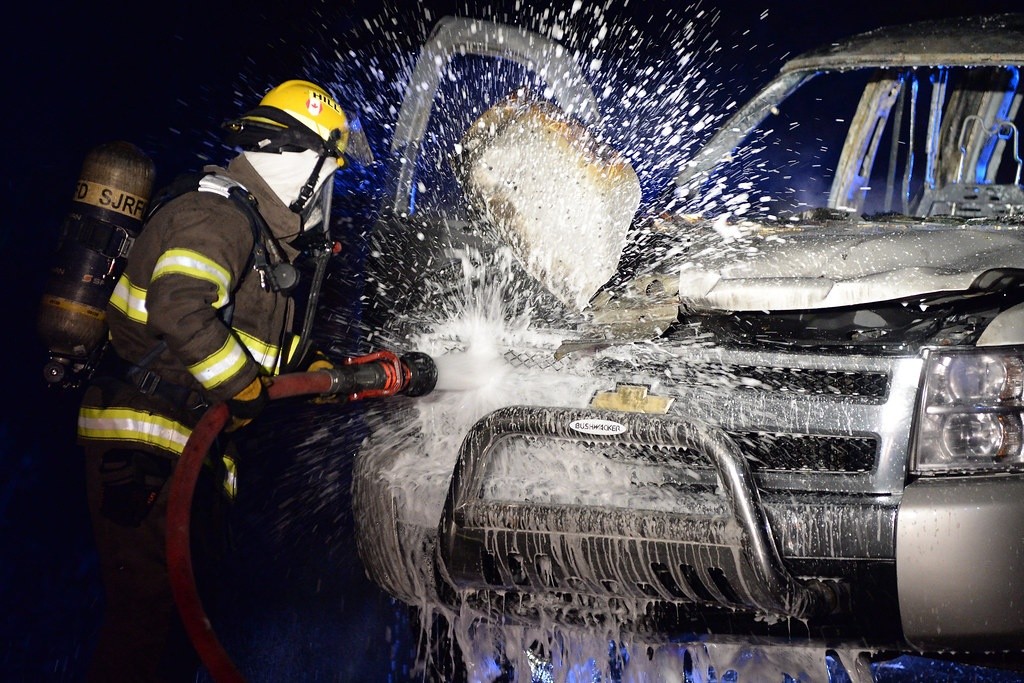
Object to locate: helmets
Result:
[221,79,350,167]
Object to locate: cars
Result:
[343,13,1024,659]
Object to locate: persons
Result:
[77,80,374,683]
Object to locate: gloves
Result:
[302,360,349,404]
[228,377,273,418]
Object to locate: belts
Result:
[107,355,207,416]
